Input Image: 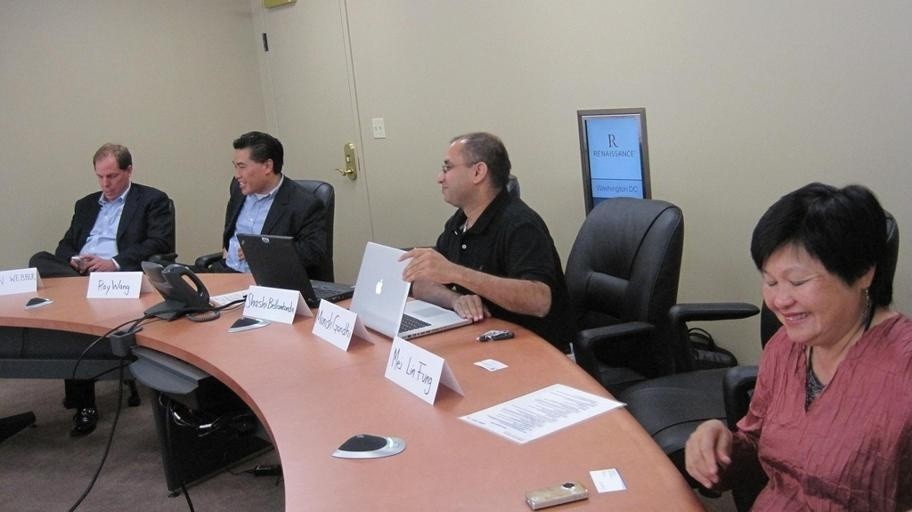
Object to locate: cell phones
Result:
[523,479,590,509]
[71,255,83,269]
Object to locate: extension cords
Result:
[254,462,280,477]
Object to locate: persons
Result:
[396,130,570,351]
[209,131,325,281]
[683,181,910,511]
[29,141,171,435]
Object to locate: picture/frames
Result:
[574,106,654,219]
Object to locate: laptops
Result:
[348,241,473,337]
[236,232,354,309]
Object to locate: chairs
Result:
[197,177,336,285]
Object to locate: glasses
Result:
[442,161,475,173]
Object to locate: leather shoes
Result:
[72,408,98,437]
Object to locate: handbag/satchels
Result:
[689,326,737,369]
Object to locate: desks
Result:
[1,272,709,511]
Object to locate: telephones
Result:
[142,260,210,309]
